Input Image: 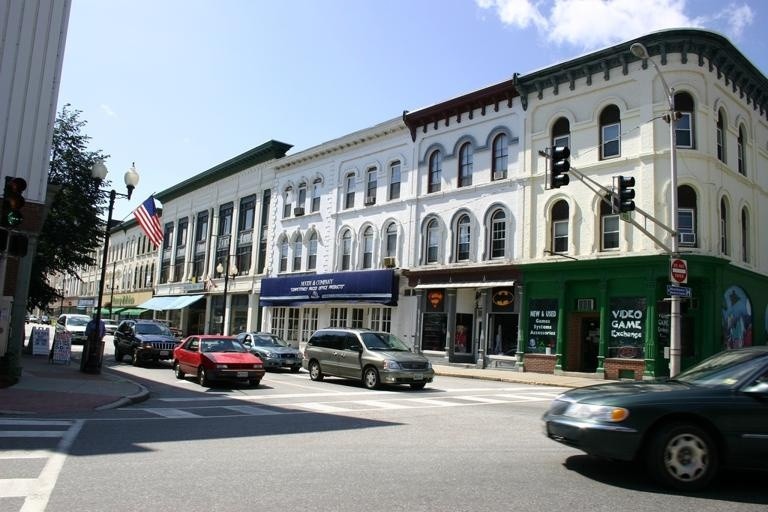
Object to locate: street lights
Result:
[216,260,239,336]
[79,154,140,376]
[106,280,120,319]
[629,42,682,379]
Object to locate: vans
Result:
[301,326,436,392]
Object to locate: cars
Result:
[170,334,266,389]
[540,344,767,492]
[25,315,51,325]
[56,313,93,344]
[100,318,118,335]
[236,331,304,374]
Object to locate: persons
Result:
[85,313,106,340]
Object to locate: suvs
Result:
[113,318,182,367]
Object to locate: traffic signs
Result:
[666,284,693,298]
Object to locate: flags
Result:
[132,194,165,248]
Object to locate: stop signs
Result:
[670,259,688,284]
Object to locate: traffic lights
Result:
[620,175,636,214]
[0,175,28,230]
[550,145,571,189]
